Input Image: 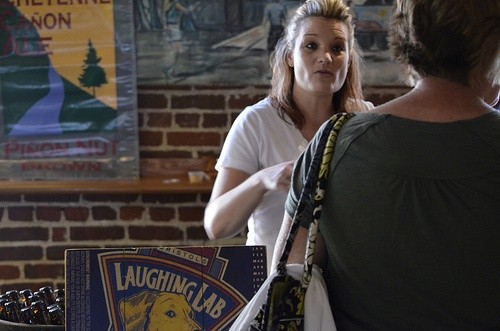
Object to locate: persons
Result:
[268,0,499,330]
[203,0,377,282]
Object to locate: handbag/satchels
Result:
[250,111,357,331]
